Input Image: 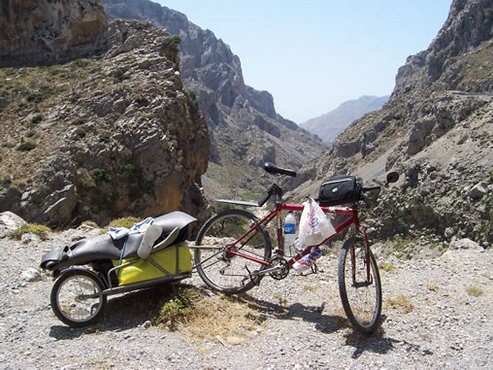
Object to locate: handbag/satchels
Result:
[111,242,193,285]
[317,175,360,207]
[298,195,336,250]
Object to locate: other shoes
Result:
[136,224,163,259]
[151,227,179,254]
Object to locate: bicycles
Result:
[194,161,399,334]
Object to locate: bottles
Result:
[292,247,323,275]
[283,211,296,245]
